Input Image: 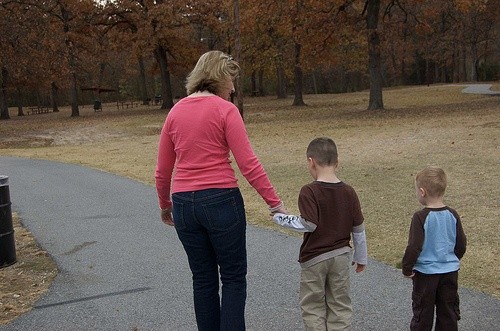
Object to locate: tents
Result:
[81,87,118,111]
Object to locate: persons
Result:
[155,49,287,331]
[403,166,466,331]
[268,137,368,331]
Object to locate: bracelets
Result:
[268,201,285,211]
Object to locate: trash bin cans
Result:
[0,175,18,267]
